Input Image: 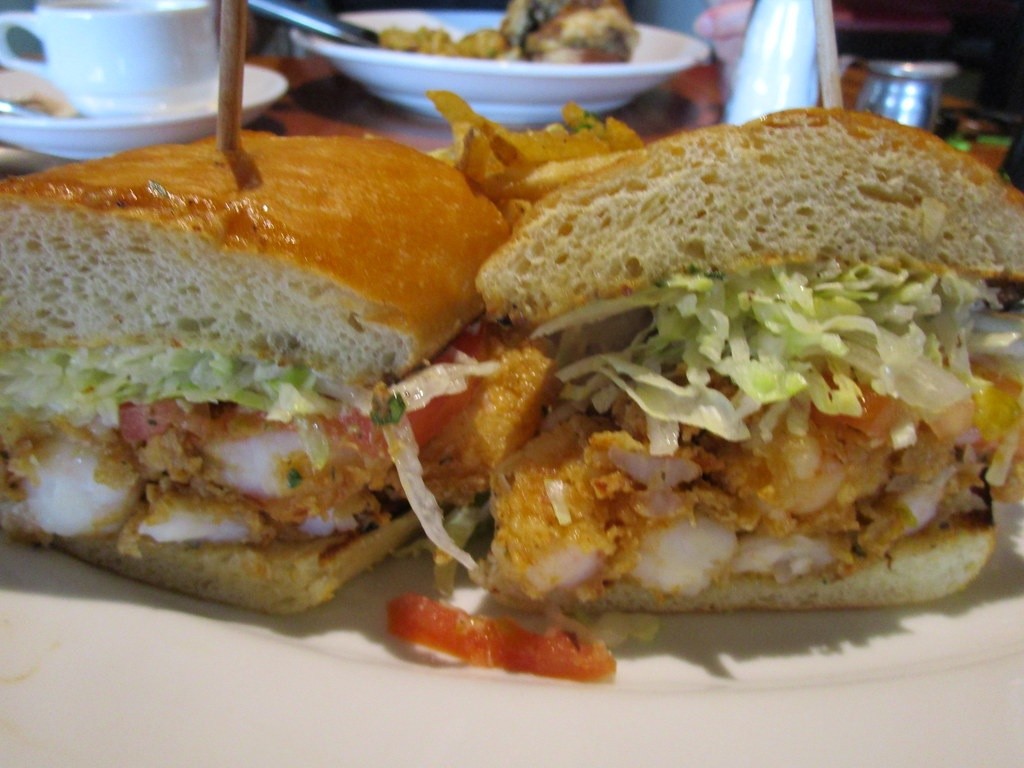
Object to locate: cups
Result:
[0,0,217,118]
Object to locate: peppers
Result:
[389,596,616,682]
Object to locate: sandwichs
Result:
[478,108,1024,608]
[0,131,509,618]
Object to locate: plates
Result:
[0,60,289,156]
[0,305,1024,767]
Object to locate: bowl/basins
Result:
[294,9,709,119]
